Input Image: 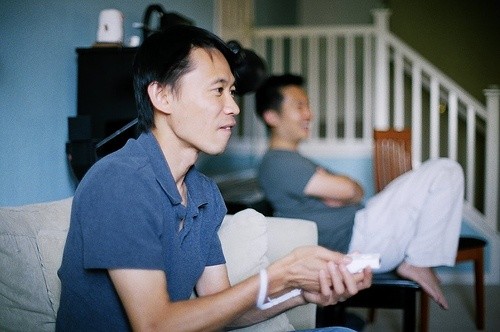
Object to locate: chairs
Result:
[319,269,430,331]
[373,129,488,331]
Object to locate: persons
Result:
[55,28,374,332]
[255,74,465,311]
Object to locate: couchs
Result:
[0,195,318,331]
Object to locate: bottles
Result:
[129,22,143,47]
[95,8,123,43]
